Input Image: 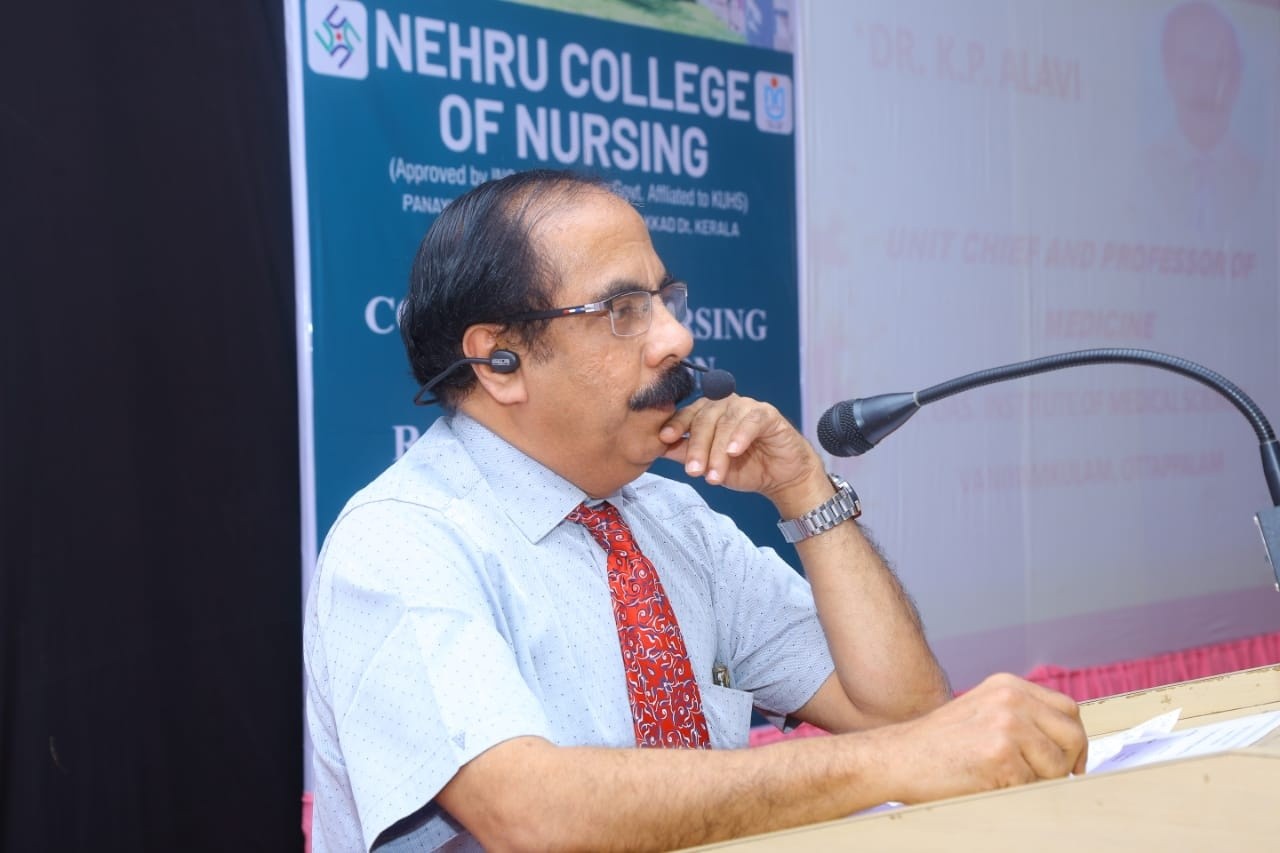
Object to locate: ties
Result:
[566,503,711,749]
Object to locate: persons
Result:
[303,171,1090,853]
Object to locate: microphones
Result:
[816,347,1279,508]
[678,360,734,400]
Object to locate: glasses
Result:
[490,282,688,337]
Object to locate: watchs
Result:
[777,472,863,545]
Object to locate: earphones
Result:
[488,350,521,374]
